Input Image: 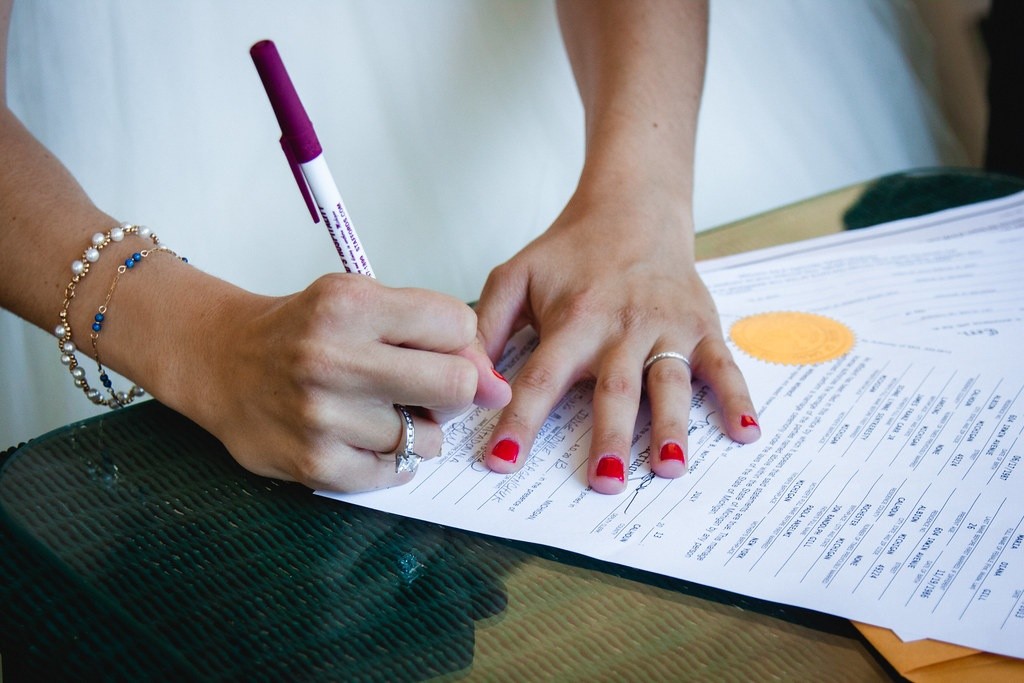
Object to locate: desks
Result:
[1,168,1024,683]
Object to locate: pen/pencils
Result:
[248,38,377,288]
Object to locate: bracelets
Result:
[52,224,188,414]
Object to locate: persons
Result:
[1,1,762,497]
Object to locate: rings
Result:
[373,404,424,475]
[641,352,694,395]
